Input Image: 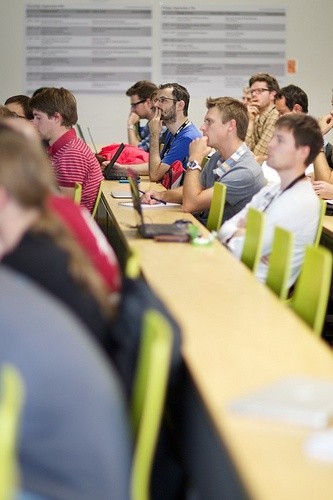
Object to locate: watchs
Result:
[186,160,202,172]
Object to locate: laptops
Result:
[76,124,98,154]
[127,168,202,239]
[103,143,128,180]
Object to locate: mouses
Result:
[174,219,193,224]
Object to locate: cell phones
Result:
[120,177,140,183]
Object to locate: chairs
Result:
[125,307,175,500]
[240,206,265,273]
[95,175,333,500]
[286,243,333,336]
[90,180,104,223]
[206,182,227,232]
[264,225,293,299]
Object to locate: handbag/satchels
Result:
[161,160,183,190]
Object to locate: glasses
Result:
[154,97,186,103]
[131,96,150,108]
[250,88,275,94]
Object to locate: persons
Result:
[0,72,333,499]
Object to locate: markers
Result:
[119,180,140,183]
[156,236,190,242]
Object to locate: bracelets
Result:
[127,124,139,131]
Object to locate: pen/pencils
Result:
[138,189,165,205]
